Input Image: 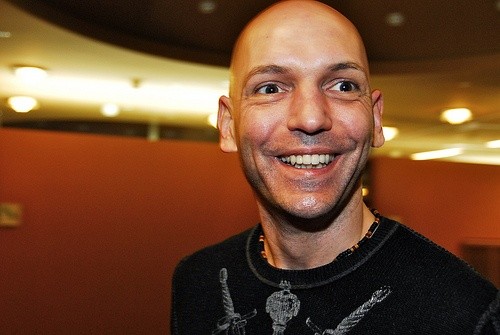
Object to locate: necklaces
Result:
[258,208,381,263]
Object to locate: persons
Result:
[170,0,500,335]
